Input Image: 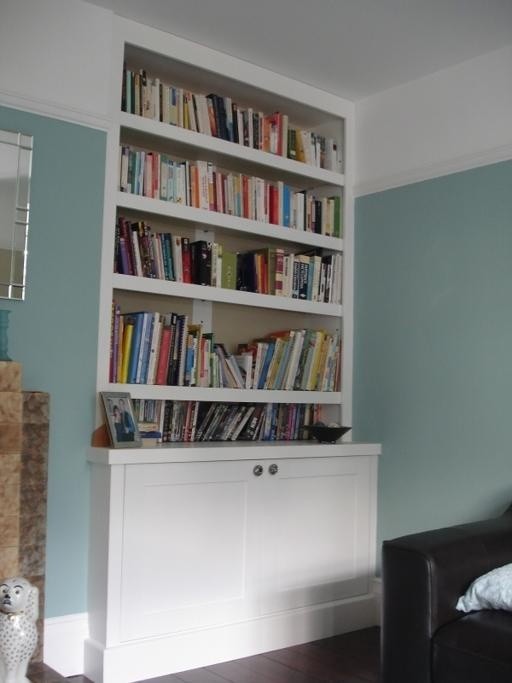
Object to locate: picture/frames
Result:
[99,392,143,448]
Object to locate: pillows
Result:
[455,563,512,613]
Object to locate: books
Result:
[130,398,326,442]
[122,70,341,175]
[111,299,342,392]
[119,145,341,239]
[115,214,342,306]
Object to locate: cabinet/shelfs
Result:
[85,18,381,683]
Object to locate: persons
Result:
[111,398,136,442]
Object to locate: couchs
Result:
[382,501,511,682]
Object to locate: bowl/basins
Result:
[302,425,352,444]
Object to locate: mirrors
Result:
[0,129,33,300]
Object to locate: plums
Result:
[315,421,325,428]
